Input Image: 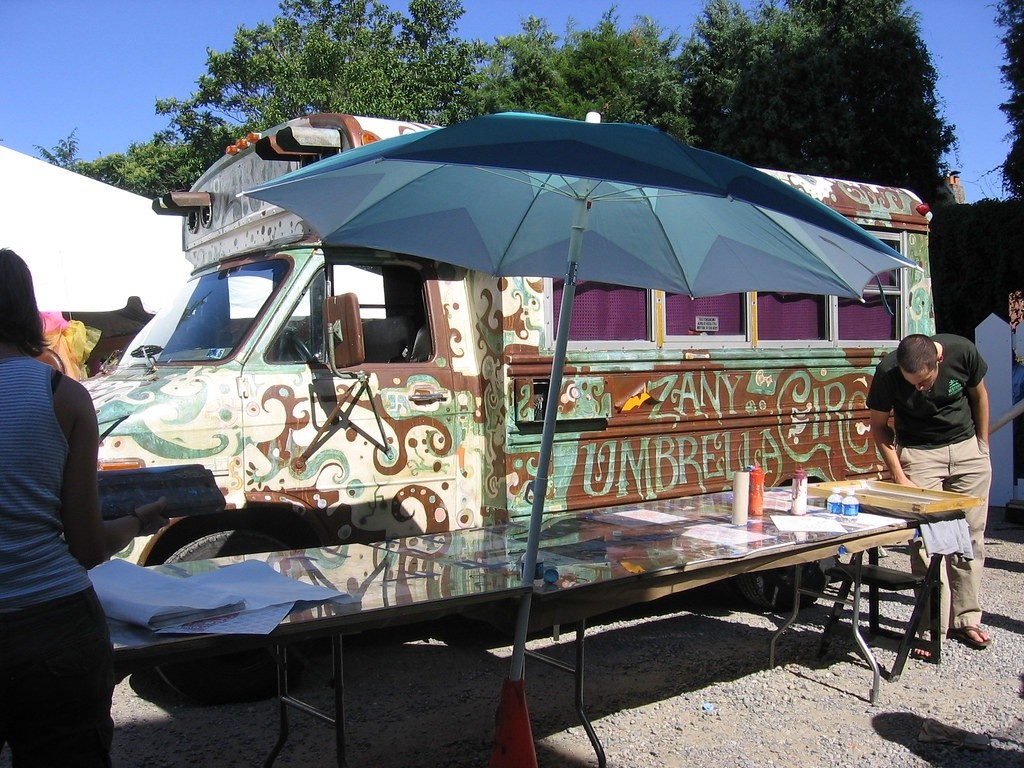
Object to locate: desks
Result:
[88,542,534,768]
[360,469,966,768]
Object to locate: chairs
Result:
[362,317,431,363]
[816,549,943,683]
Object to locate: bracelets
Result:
[125,512,144,535]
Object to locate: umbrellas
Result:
[245,109,922,681]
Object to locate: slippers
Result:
[912,648,932,662]
[949,626,992,646]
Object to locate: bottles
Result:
[521,550,545,587]
[842,490,859,523]
[791,464,808,516]
[827,488,842,519]
[748,463,765,516]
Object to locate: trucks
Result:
[72,113,938,707]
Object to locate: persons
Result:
[1012,348,1024,486]
[865,335,991,662]
[1,247,169,768]
